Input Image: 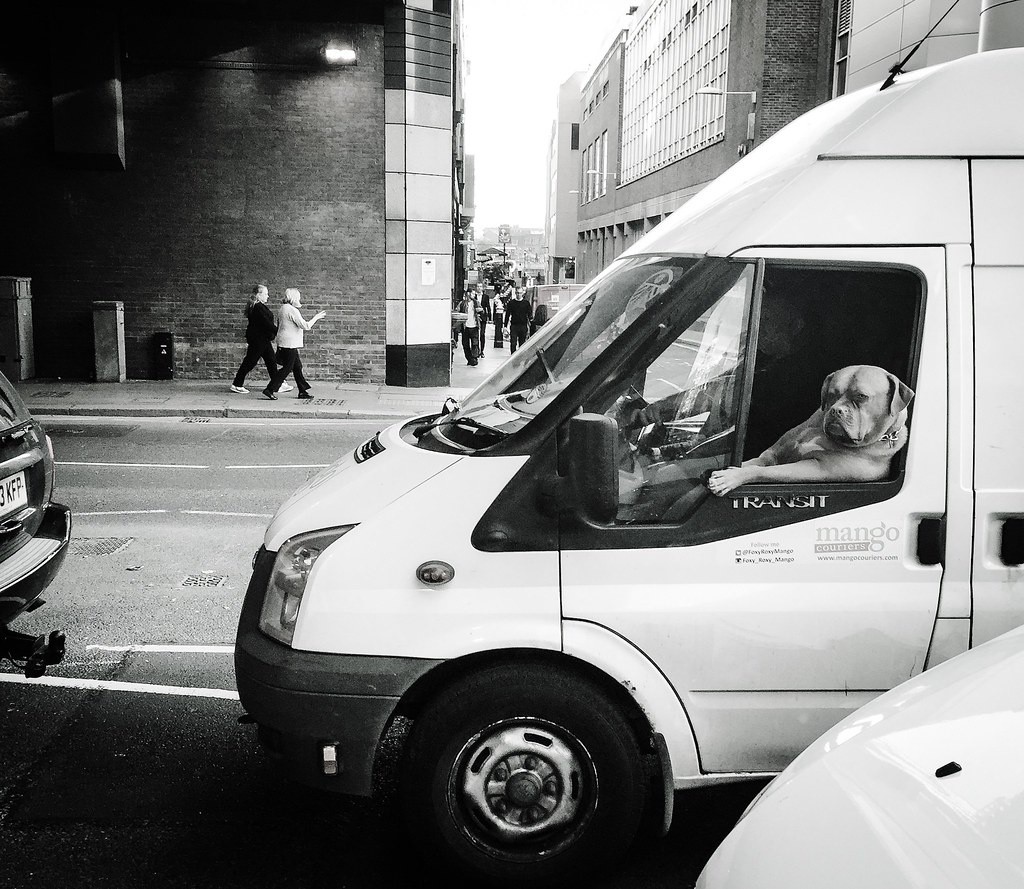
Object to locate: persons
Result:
[230,285,294,394]
[474,282,492,358]
[504,286,533,355]
[493,279,502,295]
[618,284,811,459]
[457,287,481,366]
[262,287,326,400]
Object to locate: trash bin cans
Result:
[149,331,175,380]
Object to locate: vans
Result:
[233,49,1024,873]
[0,365,65,687]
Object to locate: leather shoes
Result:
[477,354,485,358]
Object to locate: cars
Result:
[695,626,1024,889]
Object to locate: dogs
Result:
[705,363,916,498]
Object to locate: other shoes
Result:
[467,360,478,367]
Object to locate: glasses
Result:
[515,292,523,295]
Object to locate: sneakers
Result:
[262,388,278,400]
[277,380,293,393]
[298,392,314,399]
[230,384,250,394]
